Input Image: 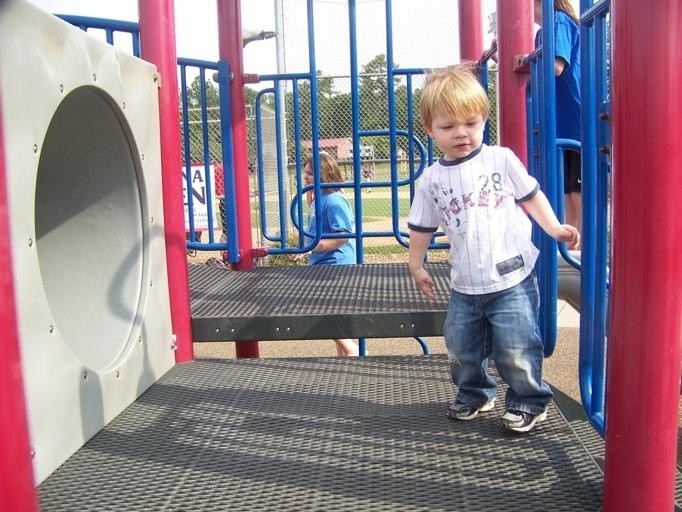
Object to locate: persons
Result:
[362,165,375,194]
[406,59,583,433]
[534,1,582,253]
[290,152,358,357]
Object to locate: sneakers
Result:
[497,406,547,435]
[445,394,494,421]
